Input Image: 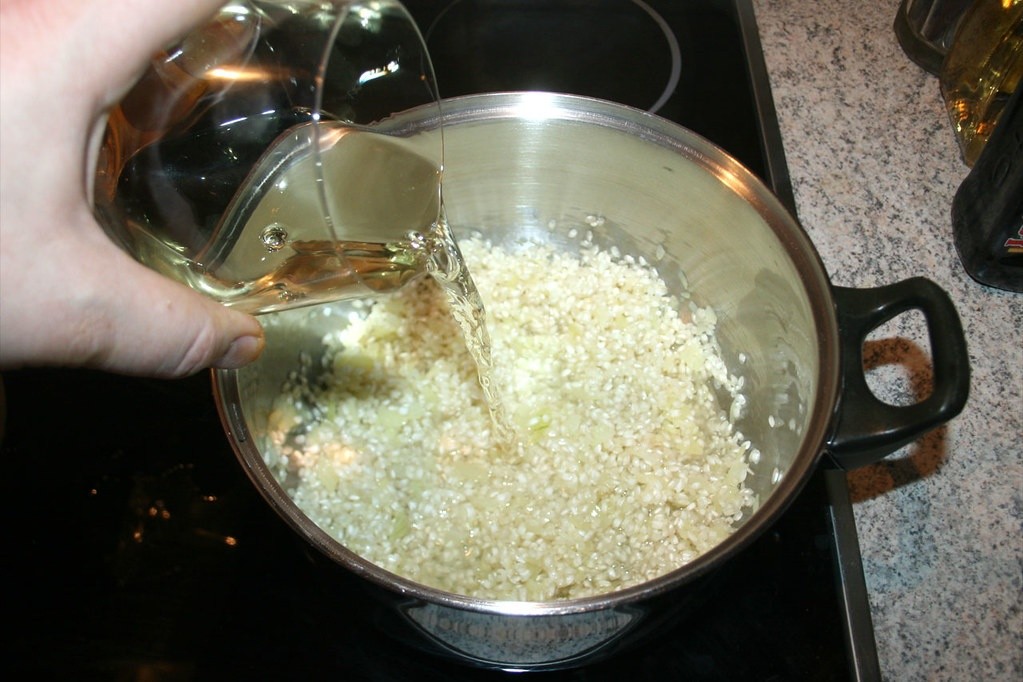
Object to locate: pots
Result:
[210,88,972,618]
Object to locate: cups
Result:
[79,0,443,319]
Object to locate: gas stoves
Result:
[0,2,891,681]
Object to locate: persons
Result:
[1,0,266,374]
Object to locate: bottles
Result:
[899,0,1022,289]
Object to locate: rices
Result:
[205,89,968,673]
